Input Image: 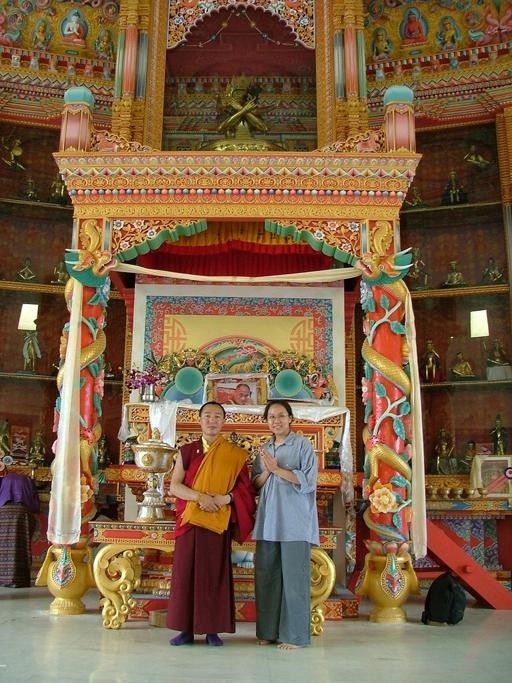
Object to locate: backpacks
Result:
[422,571,466,625]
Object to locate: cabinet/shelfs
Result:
[0,125,110,540]
[389,120,512,584]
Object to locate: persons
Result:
[164,401,257,646]
[219,383,252,405]
[439,18,458,50]
[401,144,509,476]
[399,11,427,43]
[62,10,86,45]
[30,20,49,51]
[0,137,116,470]
[96,30,116,61]
[250,399,321,650]
[196,89,287,152]
[370,30,391,60]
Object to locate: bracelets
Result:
[228,492,233,503]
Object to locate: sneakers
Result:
[206,634,223,645]
[169,632,193,644]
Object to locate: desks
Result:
[89,520,340,637]
[104,468,343,525]
[117,400,351,471]
